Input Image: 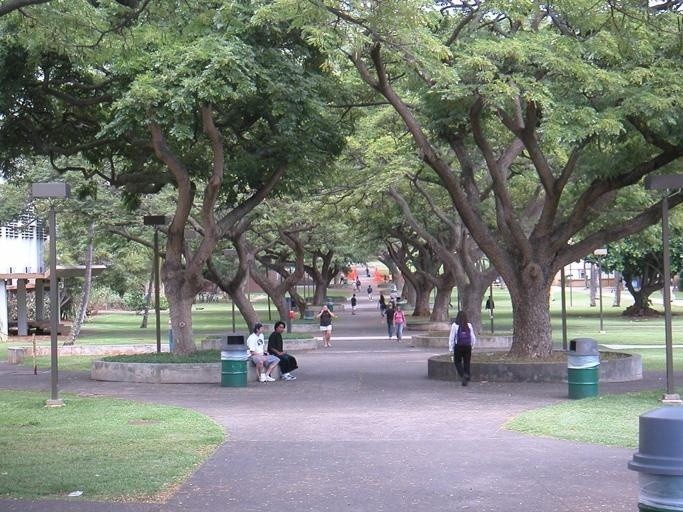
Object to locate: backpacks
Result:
[457,325,470,346]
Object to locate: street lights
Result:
[224,249,237,333]
[644,174,683,403]
[32,182,72,409]
[144,216,168,353]
[261,255,272,320]
[594,248,607,330]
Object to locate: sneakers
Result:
[462,373,470,386]
[281,373,297,381]
[259,372,276,382]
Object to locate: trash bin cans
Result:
[220,335,250,387]
[326,298,334,311]
[396,297,405,310]
[304,309,314,320]
[566,339,600,399]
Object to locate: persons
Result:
[316,304,334,348]
[351,294,357,314]
[339,264,361,293]
[485,296,494,320]
[367,285,373,301]
[448,311,476,386]
[377,289,405,343]
[365,266,369,277]
[247,322,280,382]
[268,322,298,381]
